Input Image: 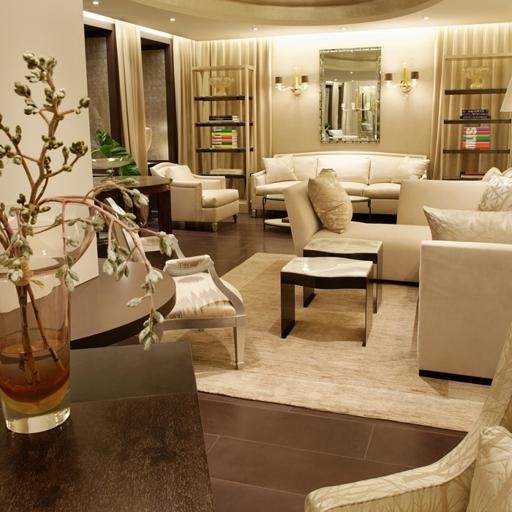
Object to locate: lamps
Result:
[500,77,512,113]
[385,69,419,93]
[275,75,310,96]
[341,101,372,112]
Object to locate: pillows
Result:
[465,427,512,512]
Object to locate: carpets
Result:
[159,252,492,433]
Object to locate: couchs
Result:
[149,162,239,233]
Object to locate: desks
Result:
[92,157,132,176]
[0,339,215,511]
[94,176,172,257]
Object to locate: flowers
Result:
[0,50,186,382]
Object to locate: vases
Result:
[0,225,71,435]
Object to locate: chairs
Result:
[104,197,246,369]
[304,328,512,512]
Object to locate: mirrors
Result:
[318,46,380,144]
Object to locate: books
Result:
[460,170,485,178]
[459,108,491,118]
[462,127,493,149]
[209,128,238,149]
[207,115,239,122]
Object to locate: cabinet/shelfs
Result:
[191,65,256,213]
[442,53,512,180]
